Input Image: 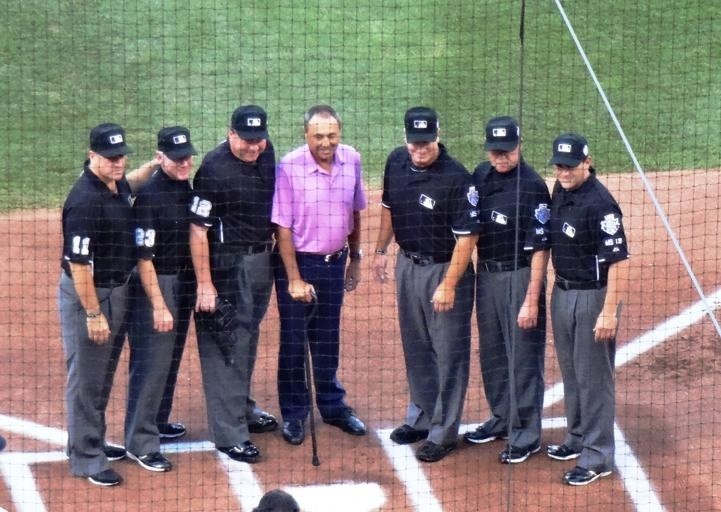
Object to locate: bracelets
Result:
[375,249,385,254]
[86,311,101,318]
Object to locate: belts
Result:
[479,259,532,277]
[93,273,131,292]
[233,243,272,254]
[157,258,202,279]
[292,246,356,266]
[396,249,461,271]
[556,276,614,297]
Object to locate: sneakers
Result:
[126,449,173,473]
[416,439,458,462]
[160,423,187,439]
[500,445,541,464]
[465,426,511,444]
[85,470,120,487]
[391,424,431,445]
[564,468,613,486]
[104,445,126,462]
[548,444,584,460]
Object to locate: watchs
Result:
[349,248,363,259]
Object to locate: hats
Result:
[156,127,199,161]
[484,117,521,152]
[405,108,440,143]
[232,106,270,142]
[544,134,589,168]
[89,124,133,159]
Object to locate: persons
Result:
[188,104,279,463]
[547,132,629,487]
[58,122,165,487]
[371,105,482,462]
[123,125,194,473]
[271,104,366,444]
[464,116,552,464]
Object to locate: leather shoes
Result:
[248,410,278,433]
[282,419,306,444]
[218,440,261,464]
[326,411,367,436]
